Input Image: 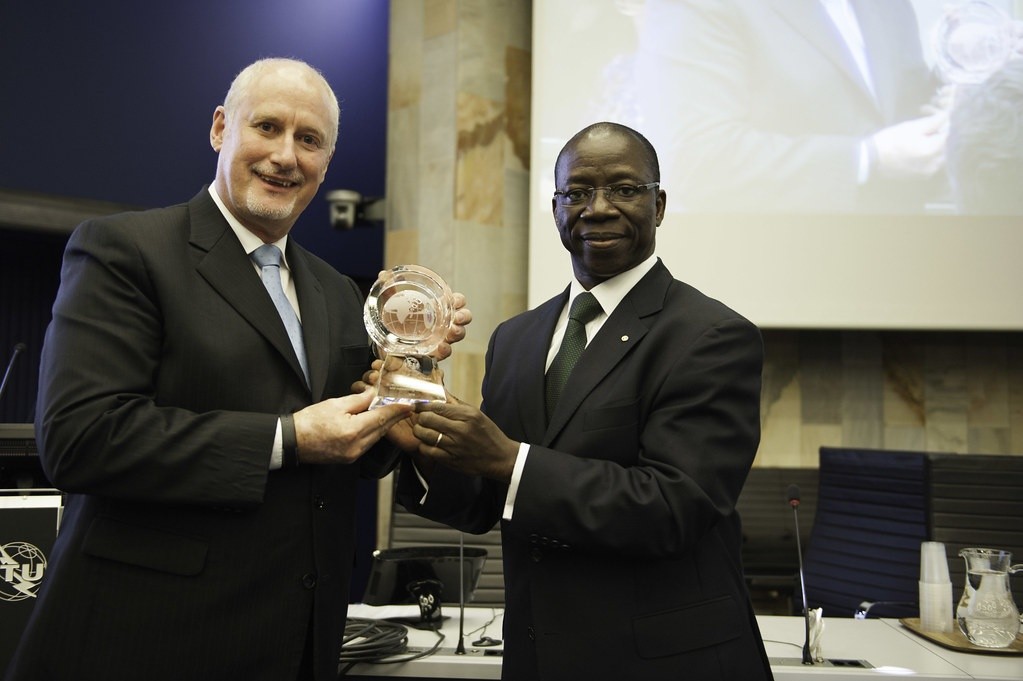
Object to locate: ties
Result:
[251,244,311,393]
[544,293,603,432]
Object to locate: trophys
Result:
[362,265,455,407]
[931,1,1016,116]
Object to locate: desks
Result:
[343,603,1023,681]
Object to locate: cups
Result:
[918,541,953,632]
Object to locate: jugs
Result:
[956,547,1023,648]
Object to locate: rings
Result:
[435,432,443,447]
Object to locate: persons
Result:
[635,1,1023,212]
[4,58,472,681]
[352,122,774,681]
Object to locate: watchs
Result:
[856,139,880,193]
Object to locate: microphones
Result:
[786,482,815,664]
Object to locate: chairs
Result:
[794,446,939,619]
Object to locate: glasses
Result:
[554,182,660,207]
